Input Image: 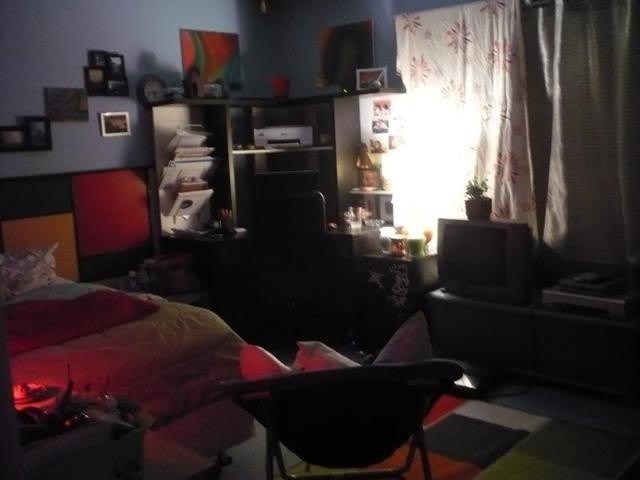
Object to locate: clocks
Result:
[138,74,170,110]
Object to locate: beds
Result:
[0,172,259,480]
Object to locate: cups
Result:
[379,225,427,258]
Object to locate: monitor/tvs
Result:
[442,221,532,304]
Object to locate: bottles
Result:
[345,205,362,223]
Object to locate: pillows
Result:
[292,340,359,371]
[372,310,432,365]
[236,339,289,382]
[0,238,62,302]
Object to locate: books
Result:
[157,121,225,228]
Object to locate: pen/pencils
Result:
[218,209,232,219]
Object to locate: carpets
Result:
[273,394,639,480]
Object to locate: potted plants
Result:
[465,180,493,221]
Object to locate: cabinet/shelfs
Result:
[425,286,640,409]
[147,96,368,232]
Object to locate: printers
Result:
[254,124,314,148]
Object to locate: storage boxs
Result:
[24,408,156,480]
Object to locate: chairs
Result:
[217,358,467,480]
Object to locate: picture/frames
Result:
[356,67,386,92]
[0,44,141,156]
